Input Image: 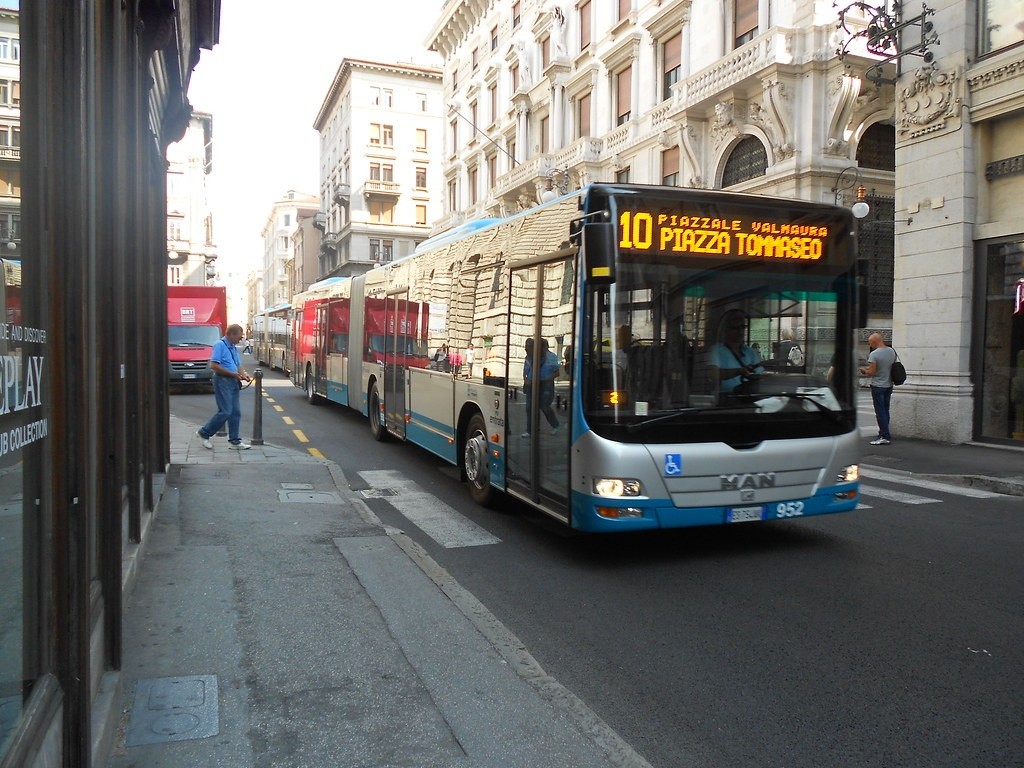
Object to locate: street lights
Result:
[541,169,568,204]
[374,251,387,269]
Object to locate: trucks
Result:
[299,301,431,370]
[167,285,228,393]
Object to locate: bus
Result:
[289,181,870,534]
[252,302,291,378]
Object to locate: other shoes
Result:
[521,432,530,437]
[469,375,472,379]
[551,426,560,435]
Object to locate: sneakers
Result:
[870,437,890,445]
[196,431,212,448]
[228,442,252,450]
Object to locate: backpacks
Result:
[786,342,801,367]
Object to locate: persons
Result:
[827,353,835,382]
[196,324,252,450]
[706,309,763,394]
[242,338,254,354]
[858,332,896,445]
[520,338,562,437]
[770,327,803,367]
[751,343,765,363]
[434,343,476,378]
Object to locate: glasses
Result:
[729,324,746,330]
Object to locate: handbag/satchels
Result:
[891,348,907,385]
[239,381,242,390]
[523,381,527,394]
